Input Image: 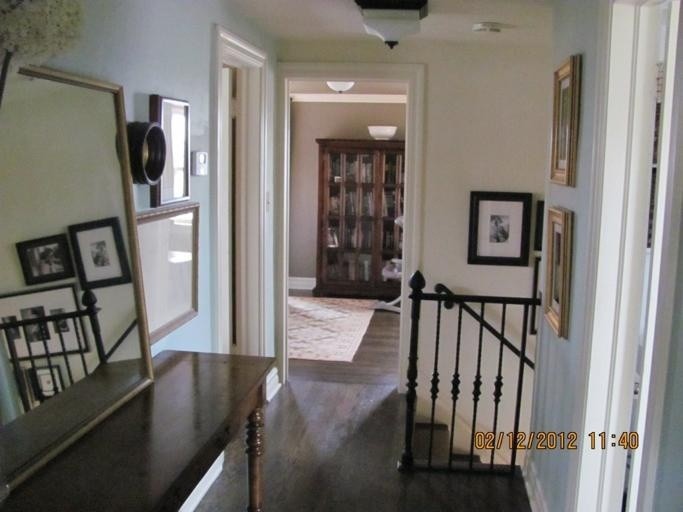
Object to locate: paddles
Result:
[287,295,379,363]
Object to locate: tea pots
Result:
[0,63,155,503]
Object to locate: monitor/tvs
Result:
[328,151,404,282]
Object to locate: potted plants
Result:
[28,364,64,400]
[467,190,532,266]
[135,201,200,345]
[533,199,543,252]
[15,234,75,285]
[0,285,90,361]
[150,94,191,208]
[530,257,542,335]
[543,205,574,339]
[548,54,582,187]
[67,216,132,290]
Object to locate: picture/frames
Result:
[0,350,277,512]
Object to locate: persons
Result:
[94,240,110,266]
[490,216,509,242]
[39,249,57,275]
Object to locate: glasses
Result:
[355,0,429,51]
[327,81,354,93]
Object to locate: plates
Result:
[311,138,405,302]
